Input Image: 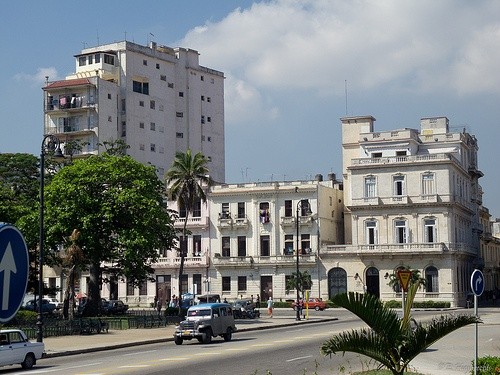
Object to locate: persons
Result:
[267,297,274,318]
[299,297,304,314]
[255,294,261,308]
[154,294,179,313]
[223,297,228,303]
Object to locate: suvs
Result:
[173,303,237,345]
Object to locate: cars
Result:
[292,297,326,311]
[0,329,45,370]
[22,298,129,317]
[169,294,253,318]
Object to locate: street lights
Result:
[295,200,313,321]
[36,134,64,342]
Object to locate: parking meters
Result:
[306,289,311,320]
